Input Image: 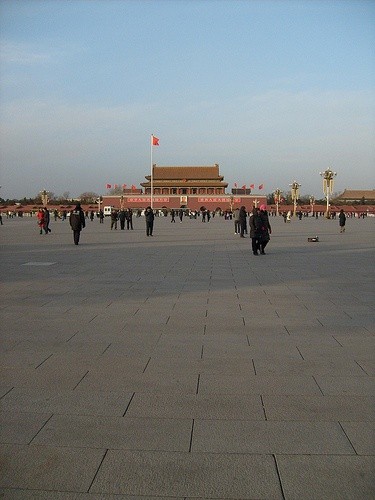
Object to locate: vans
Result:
[103,205,114,218]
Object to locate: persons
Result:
[69,203,86,246]
[219,205,368,255]
[145,206,154,237]
[0,203,216,245]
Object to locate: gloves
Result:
[269,229,271,234]
[262,226,265,230]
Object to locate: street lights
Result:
[228,194,234,211]
[272,186,283,216]
[38,188,51,207]
[288,178,302,219]
[320,166,337,220]
[308,194,316,217]
[252,198,260,208]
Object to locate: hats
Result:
[260,204,266,210]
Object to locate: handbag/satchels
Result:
[38,221,42,224]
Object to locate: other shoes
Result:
[261,252,265,254]
[238,233,240,234]
[40,231,42,234]
[235,233,236,234]
[49,229,51,232]
[253,252,258,255]
[240,235,245,238]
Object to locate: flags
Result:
[242,185,247,190]
[131,185,136,191]
[150,133,159,146]
[258,184,264,189]
[114,184,118,188]
[106,184,111,189]
[250,184,255,188]
[122,184,128,190]
[234,182,237,187]
